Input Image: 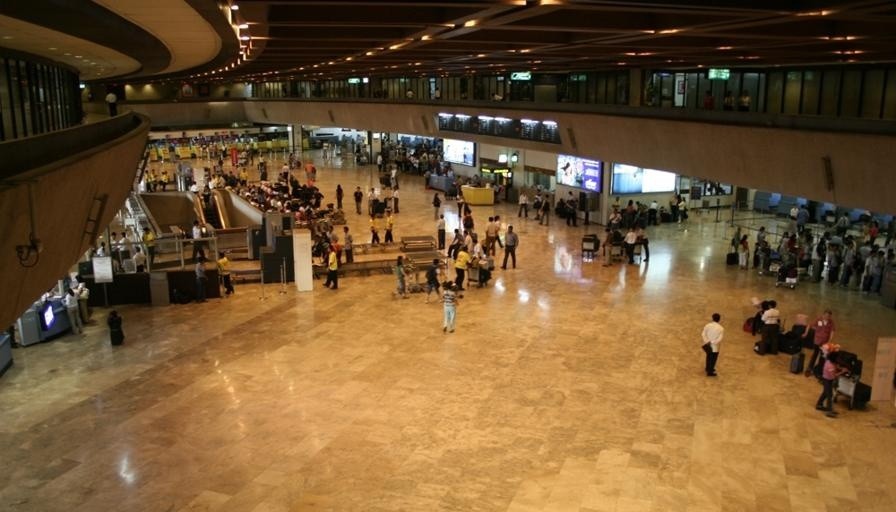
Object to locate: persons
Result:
[492,93,504,100]
[703,89,716,109]
[647,93,656,106]
[722,90,735,110]
[104,90,117,116]
[405,89,414,99]
[738,90,751,112]
[373,88,387,100]
[434,87,441,99]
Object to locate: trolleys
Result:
[380,174,392,191]
[292,157,301,169]
[831,373,874,409]
[465,262,492,289]
[369,197,384,218]
[776,267,800,288]
[408,265,431,294]
[580,235,600,257]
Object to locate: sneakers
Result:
[602,262,614,268]
[391,291,409,300]
[499,264,517,272]
[628,258,635,264]
[816,403,840,419]
[518,215,551,227]
[424,298,445,304]
[443,325,455,335]
[321,280,339,292]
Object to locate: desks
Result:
[428,173,454,193]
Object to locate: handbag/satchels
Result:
[703,344,714,354]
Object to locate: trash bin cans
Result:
[149,271,170,307]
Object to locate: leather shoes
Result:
[705,366,718,377]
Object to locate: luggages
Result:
[790,354,805,374]
[727,252,739,266]
[738,251,750,270]
